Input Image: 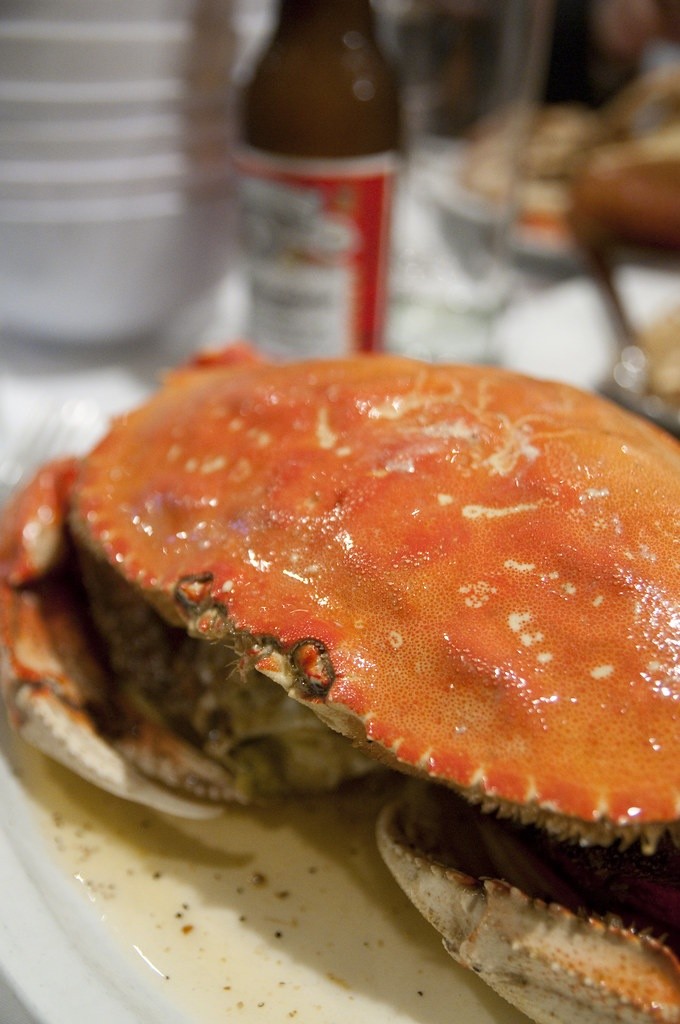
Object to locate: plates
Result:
[0,490,540,1024]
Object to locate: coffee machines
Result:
[231,0,410,379]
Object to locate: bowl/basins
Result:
[0,1,247,353]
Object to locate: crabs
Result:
[0,348,680,1024]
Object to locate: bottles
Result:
[364,1,558,370]
